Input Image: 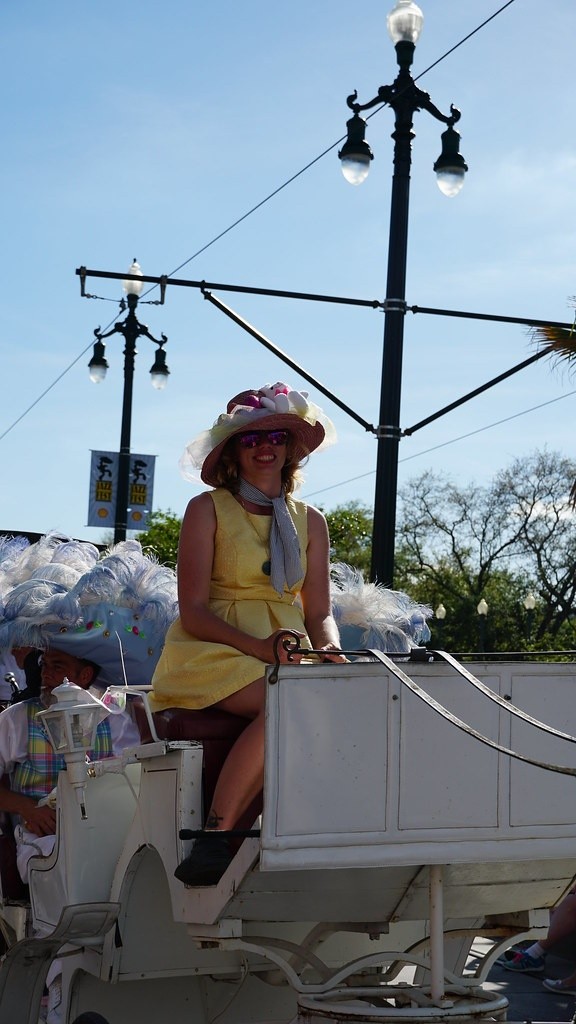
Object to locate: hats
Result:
[182,381,335,488]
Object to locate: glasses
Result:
[232,425,293,449]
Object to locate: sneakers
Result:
[503,942,545,971]
[174,835,237,887]
[543,974,576,996]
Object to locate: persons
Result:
[505,891,575,995]
[0,644,143,897]
[149,380,349,888]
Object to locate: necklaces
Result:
[243,502,274,576]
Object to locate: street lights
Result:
[335,2,471,592]
[85,257,173,548]
